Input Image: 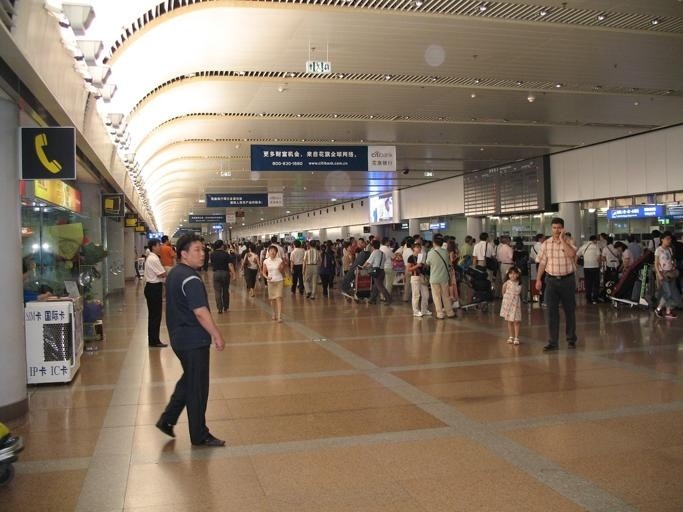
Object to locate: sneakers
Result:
[272,312,282,323]
[654,309,679,320]
[413,311,457,320]
[568,342,575,349]
[507,337,521,344]
[306,292,314,300]
[543,343,553,351]
[219,307,228,313]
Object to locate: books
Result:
[47,295,59,301]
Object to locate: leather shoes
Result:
[156,420,174,437]
[201,435,225,446]
[151,343,169,347]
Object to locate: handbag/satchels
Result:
[283,274,293,287]
[578,255,584,265]
[663,270,679,281]
[420,273,430,285]
[486,257,497,270]
[369,267,379,277]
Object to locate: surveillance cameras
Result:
[402,170,410,174]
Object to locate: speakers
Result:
[364,227,370,233]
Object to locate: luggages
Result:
[631,280,641,301]
[458,267,491,304]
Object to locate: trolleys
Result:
[450,267,487,313]
[340,266,372,302]
[606,264,650,310]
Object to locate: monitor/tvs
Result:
[401,223,408,230]
[430,224,439,230]
[420,222,430,231]
[440,223,448,229]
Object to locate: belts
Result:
[549,273,574,280]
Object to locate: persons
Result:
[396,230,682,318]
[156,234,227,446]
[231,234,396,322]
[23,258,52,302]
[535,218,579,351]
[135,236,236,348]
[500,266,523,344]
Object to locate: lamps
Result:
[44,4,158,233]
